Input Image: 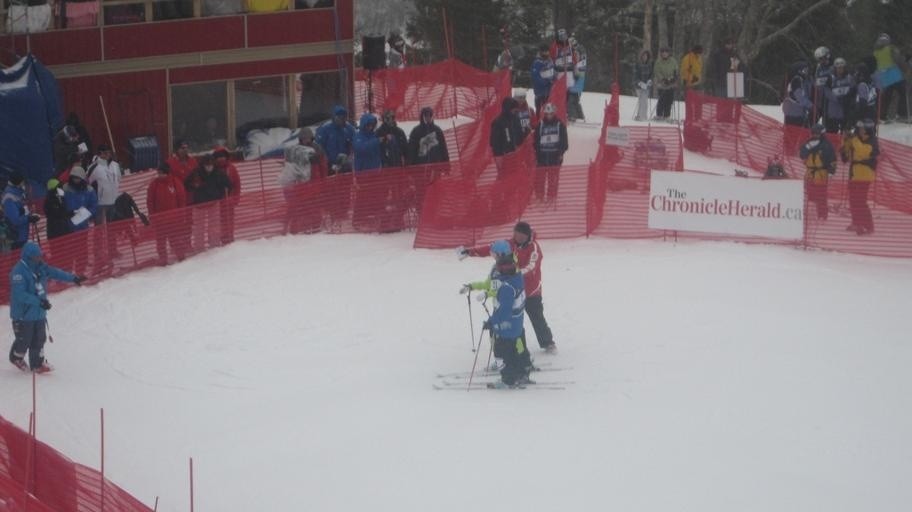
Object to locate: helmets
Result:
[490,239,512,257]
[857,118,875,134]
[495,256,516,274]
[812,123,826,137]
[542,102,555,113]
[814,47,846,69]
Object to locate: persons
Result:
[1,111,239,280]
[711,38,745,121]
[282,106,451,234]
[781,47,877,157]
[872,33,911,124]
[800,122,839,219]
[488,90,569,211]
[680,45,704,121]
[382,31,414,120]
[10,237,87,374]
[529,29,588,124]
[653,47,678,120]
[482,257,536,390]
[458,239,529,370]
[505,223,557,352]
[631,49,652,121]
[835,117,879,233]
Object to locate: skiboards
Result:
[434,365,576,391]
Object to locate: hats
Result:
[514,222,531,235]
